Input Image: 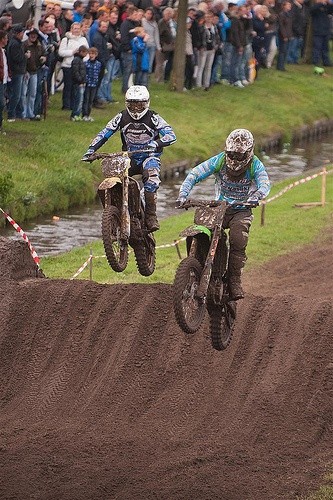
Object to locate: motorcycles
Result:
[173,199,260,351]
[80,148,157,277]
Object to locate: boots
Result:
[144,191,159,231]
[228,253,244,298]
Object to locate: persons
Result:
[82,85,177,241]
[174,129,271,301]
[0,0,333,134]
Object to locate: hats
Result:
[26,29,39,38]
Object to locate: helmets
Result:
[225,128,254,171]
[124,85,151,120]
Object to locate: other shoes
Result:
[98,99,107,104]
[83,117,93,121]
[233,81,244,88]
[243,80,249,85]
[72,116,81,121]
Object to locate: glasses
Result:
[126,101,145,112]
[227,151,247,161]
